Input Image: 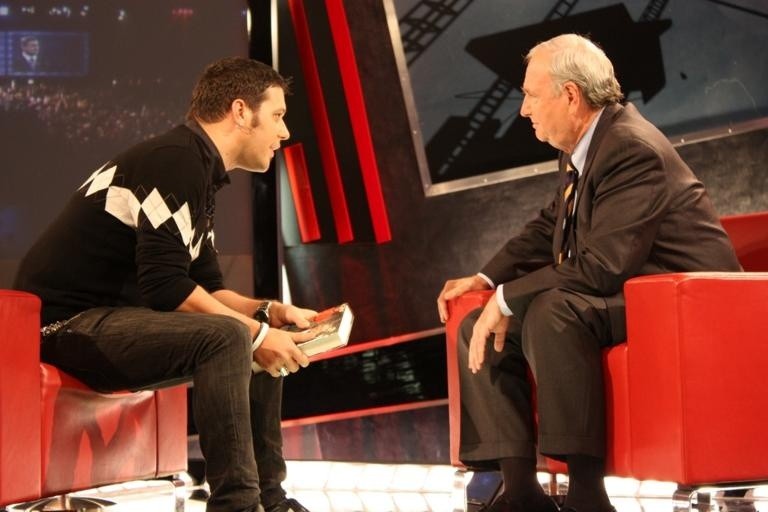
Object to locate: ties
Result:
[558,155,578,264]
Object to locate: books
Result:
[251,303,354,375]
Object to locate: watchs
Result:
[253,300,274,326]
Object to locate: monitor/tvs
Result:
[382,0,768,199]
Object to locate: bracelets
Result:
[252,321,270,353]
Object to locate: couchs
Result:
[0,288,193,508]
[443,210,767,510]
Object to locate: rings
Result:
[277,365,289,378]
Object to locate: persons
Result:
[435,34,743,511]
[11,57,319,512]
[16,36,45,72]
